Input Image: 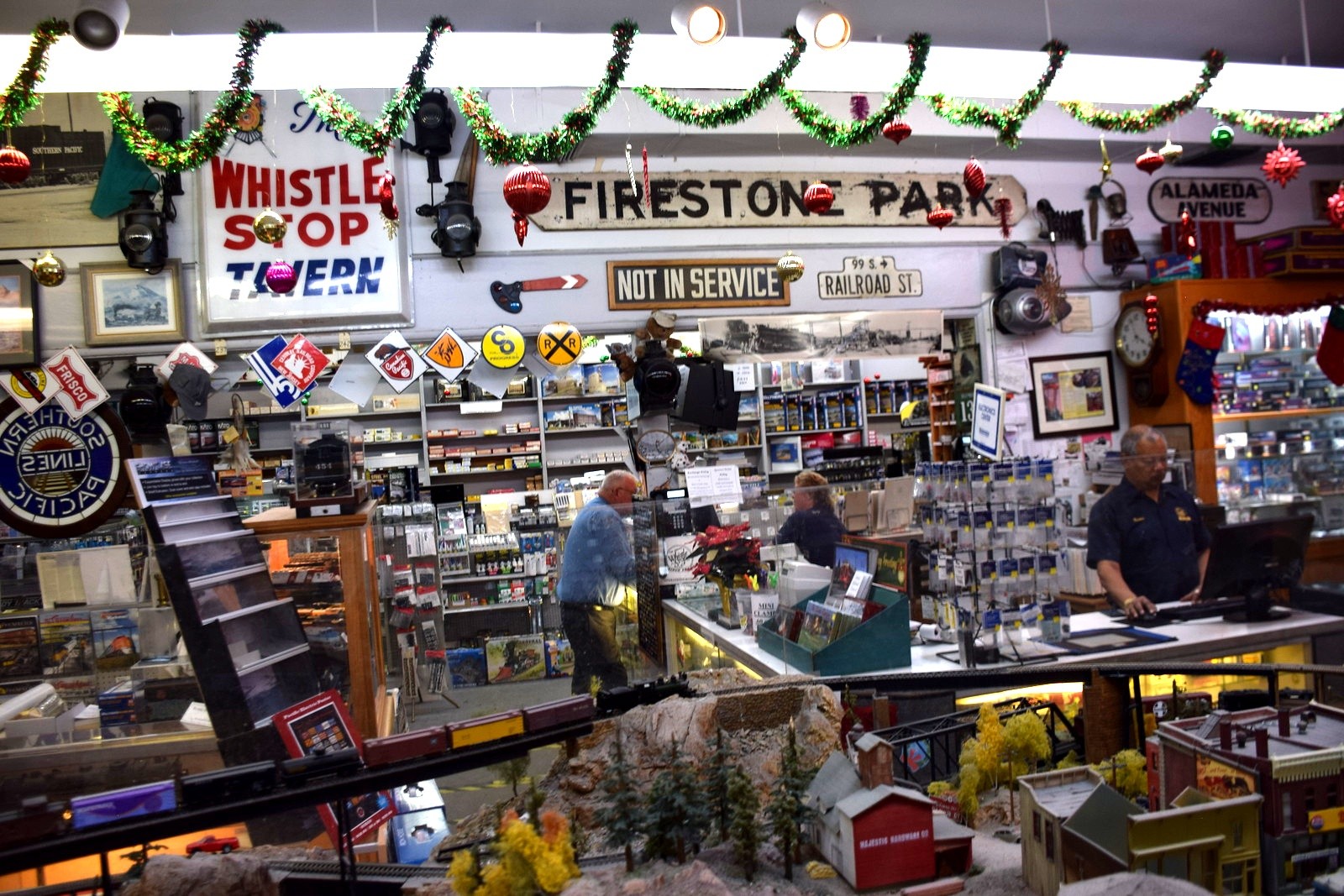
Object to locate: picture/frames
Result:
[79,258,187,347]
[0,258,40,370]
[1152,423,1195,480]
[1028,350,1119,440]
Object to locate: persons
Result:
[1086,426,1214,620]
[765,471,846,568]
[555,469,641,693]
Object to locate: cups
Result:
[735,590,779,636]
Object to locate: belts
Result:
[560,601,614,611]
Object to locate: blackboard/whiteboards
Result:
[630,497,665,670]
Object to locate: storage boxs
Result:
[1160,220,1344,280]
[394,778,445,820]
[389,808,449,866]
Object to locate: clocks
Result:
[1115,301,1169,407]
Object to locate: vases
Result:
[704,574,749,620]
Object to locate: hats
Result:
[170,364,209,422]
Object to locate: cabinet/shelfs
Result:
[1120,281,1344,589]
[1,349,969,852]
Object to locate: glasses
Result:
[611,487,636,495]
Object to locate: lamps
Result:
[1100,179,1132,228]
[671,0,727,47]
[118,97,184,273]
[796,0,851,51]
[401,87,481,260]
[70,0,131,51]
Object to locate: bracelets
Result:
[1123,597,1135,608]
[1194,588,1200,594]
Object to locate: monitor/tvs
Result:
[831,541,880,580]
[1198,513,1314,621]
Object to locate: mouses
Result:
[1132,610,1157,622]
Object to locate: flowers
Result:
[688,524,763,585]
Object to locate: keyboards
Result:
[1156,597,1244,621]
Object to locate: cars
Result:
[186,834,240,855]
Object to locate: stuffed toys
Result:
[607,309,682,382]
